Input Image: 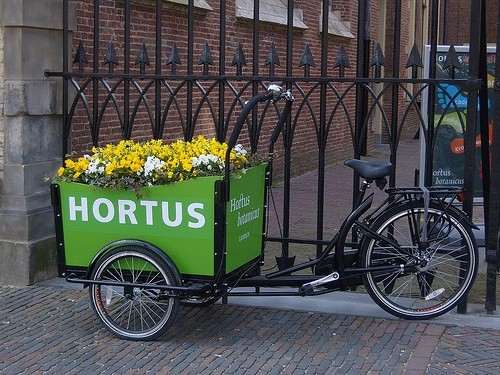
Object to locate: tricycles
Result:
[48,84,480,343]
